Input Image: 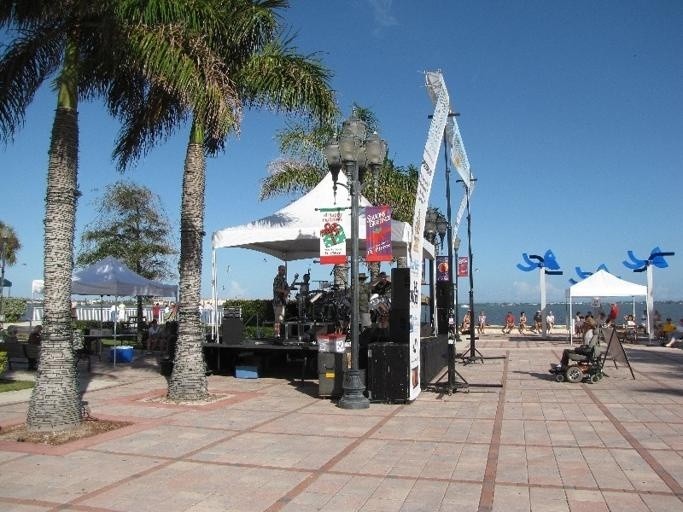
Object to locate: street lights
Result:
[452,233,462,345]
[424,209,449,339]
[323,104,391,414]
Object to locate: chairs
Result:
[623,325,638,344]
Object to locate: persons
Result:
[463,310,471,328]
[28,325,42,366]
[145,303,177,354]
[575,302,683,347]
[503,312,515,333]
[359,273,372,332]
[534,311,542,333]
[300,274,310,292]
[550,316,597,372]
[372,272,392,295]
[546,311,554,333]
[5,325,36,369]
[478,311,486,334]
[273,266,292,338]
[110,302,125,322]
[519,312,526,334]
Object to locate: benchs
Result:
[0,342,91,371]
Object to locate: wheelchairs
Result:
[553,327,606,385]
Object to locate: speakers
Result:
[391,268,409,310]
[389,308,409,344]
[437,307,449,334]
[437,280,451,307]
